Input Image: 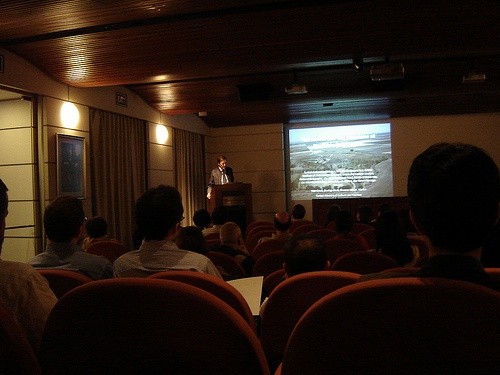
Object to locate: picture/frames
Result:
[54,132,88,198]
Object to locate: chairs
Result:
[0,220,500,375]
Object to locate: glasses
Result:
[177,216,185,227]
[83,216,88,222]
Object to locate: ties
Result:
[218,168,227,184]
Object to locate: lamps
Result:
[460,54,486,86]
[368,58,406,81]
[284,69,308,95]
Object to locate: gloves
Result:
[207,193,211,199]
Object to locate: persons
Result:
[26,195,113,280]
[260,233,331,315]
[84,203,500,271]
[406,142,500,291]
[111,185,223,279]
[0,178,58,358]
[205,155,235,200]
